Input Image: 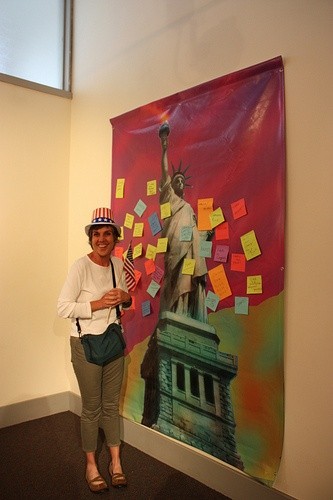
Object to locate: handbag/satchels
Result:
[81,323,126,367]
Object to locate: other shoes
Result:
[108,464,127,488]
[85,471,108,493]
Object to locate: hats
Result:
[86,208,121,237]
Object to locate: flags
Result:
[123,244,137,293]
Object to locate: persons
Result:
[55,207,132,492]
[157,120,210,324]
